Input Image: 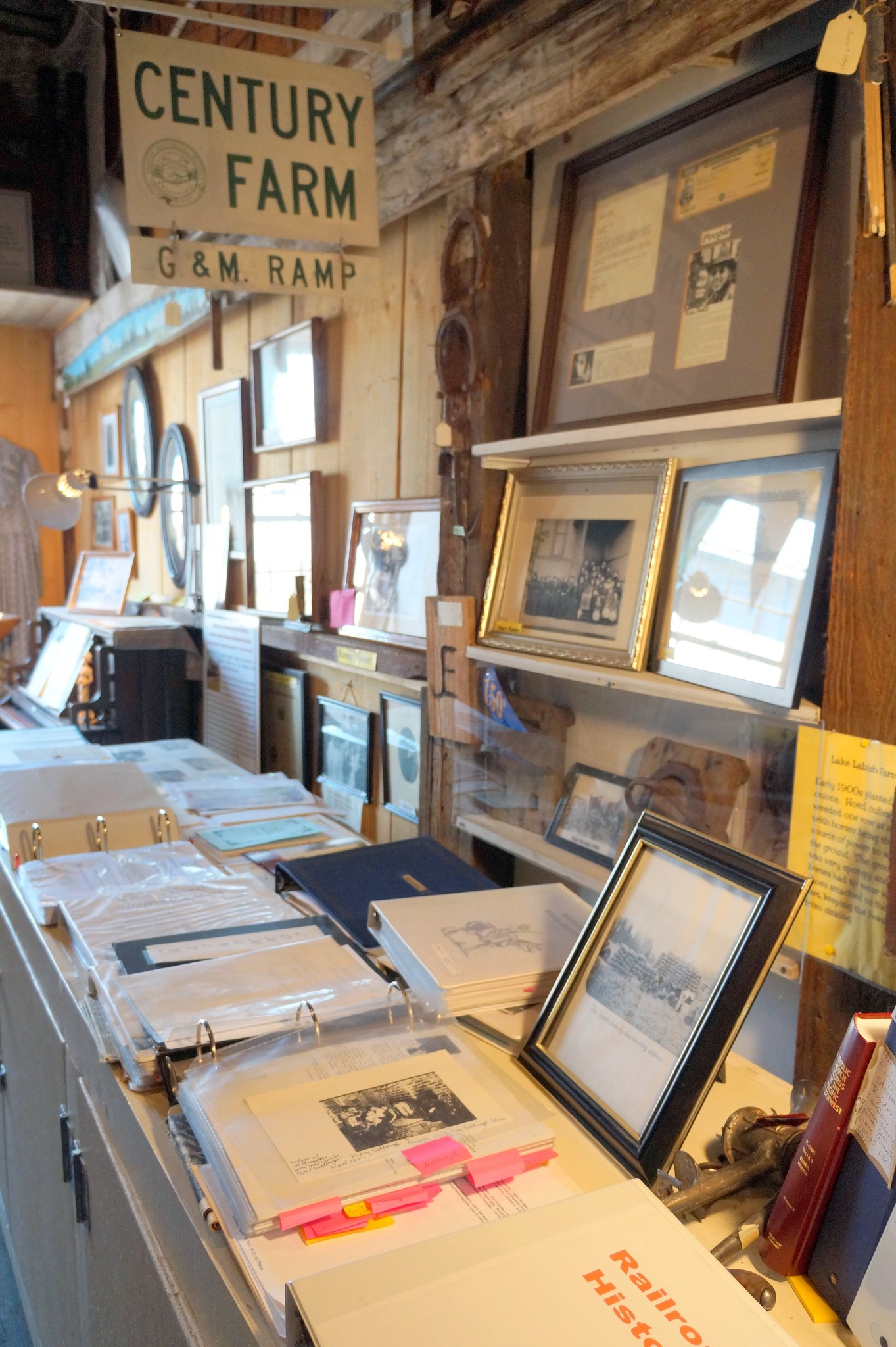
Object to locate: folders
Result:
[275,836,503,948]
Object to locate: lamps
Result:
[20,466,200,530]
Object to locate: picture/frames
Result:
[63,550,136,617]
[375,692,425,827]
[100,413,123,479]
[260,663,312,790]
[159,416,194,590]
[513,800,813,1194]
[309,694,375,806]
[251,315,327,453]
[113,508,136,574]
[86,496,115,550]
[544,760,633,872]
[112,363,158,518]
[333,497,443,654]
[647,449,841,709]
[474,457,682,674]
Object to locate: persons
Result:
[524,559,622,626]
[705,254,735,303]
[572,350,593,384]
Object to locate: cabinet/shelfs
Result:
[0,860,275,1345]
[452,410,890,1098]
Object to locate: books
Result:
[0,736,896,1347]
